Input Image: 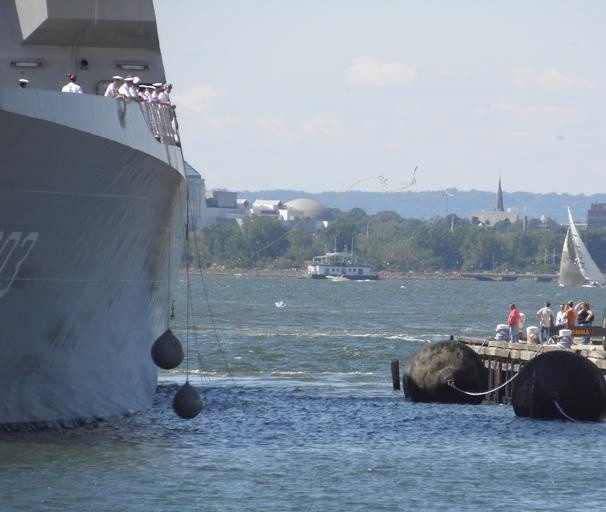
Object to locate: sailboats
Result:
[559,204,606,289]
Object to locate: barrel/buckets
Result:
[526,327,539,345]
[559,330,571,347]
[496,324,510,341]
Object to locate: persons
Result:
[574,301,595,345]
[508,303,523,343]
[61,71,82,93]
[18,78,29,88]
[562,300,577,332]
[104,75,174,106]
[555,304,569,333]
[536,302,555,344]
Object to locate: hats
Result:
[133,77,141,84]
[163,82,173,88]
[152,83,163,88]
[138,84,147,88]
[124,78,134,83]
[144,85,156,90]
[113,76,123,81]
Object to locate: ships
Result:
[0,1,193,426]
